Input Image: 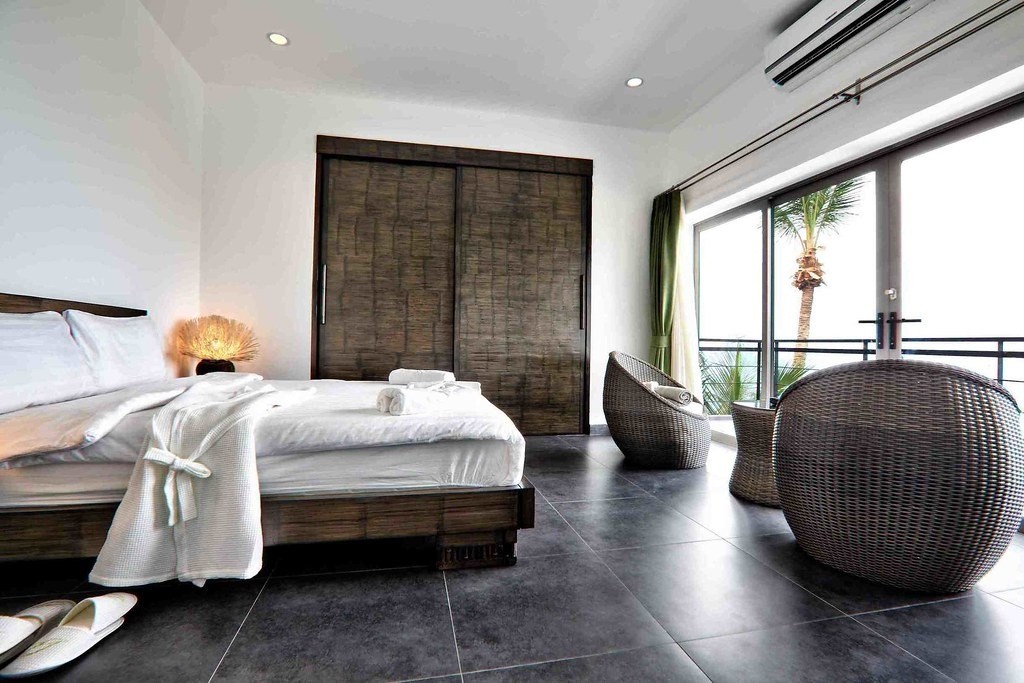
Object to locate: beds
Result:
[0,292,535,587]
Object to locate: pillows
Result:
[0,309,102,415]
[63,309,178,392]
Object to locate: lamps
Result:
[177,314,259,376]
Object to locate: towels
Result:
[641,381,693,407]
[376,368,482,416]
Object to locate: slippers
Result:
[0,593,138,677]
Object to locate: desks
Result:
[727,402,781,508]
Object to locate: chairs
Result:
[771,358,1024,594]
[603,352,711,470]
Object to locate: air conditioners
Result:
[762,0,933,93]
